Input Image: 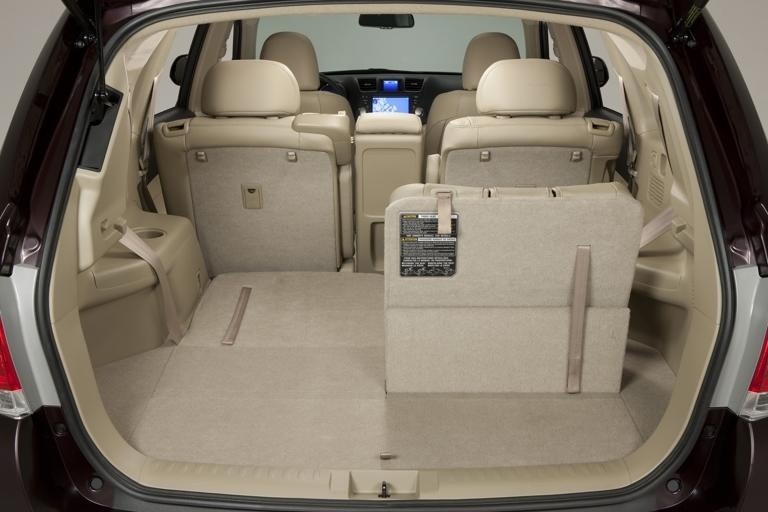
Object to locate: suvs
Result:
[2,0,766,511]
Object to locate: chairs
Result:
[151,30,645,395]
